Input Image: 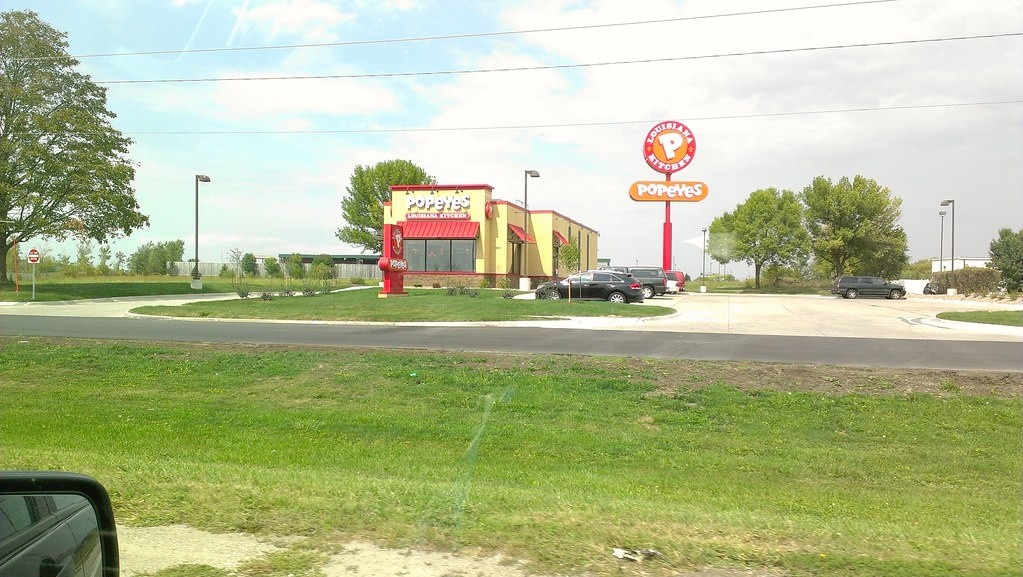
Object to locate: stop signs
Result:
[26,248,40,263]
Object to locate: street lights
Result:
[193,175,211,280]
[939,200,955,272]
[520,170,540,277]
[702,228,707,286]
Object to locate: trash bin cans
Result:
[518,277,531,291]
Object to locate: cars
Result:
[923,283,943,294]
[536,271,645,303]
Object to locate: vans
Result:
[830,275,906,299]
[599,266,685,298]
